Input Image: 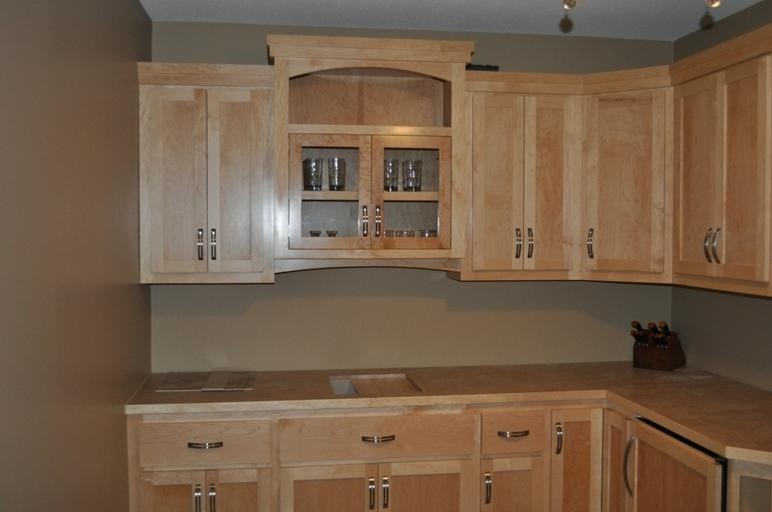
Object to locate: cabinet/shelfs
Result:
[281,394,480,511]
[671,27,772,299]
[267,62,475,272]
[137,63,274,283]
[481,391,606,510]
[446,66,670,285]
[124,401,280,512]
[606,392,771,512]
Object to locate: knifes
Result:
[630,320,672,352]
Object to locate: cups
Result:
[383,229,437,238]
[302,157,324,192]
[327,156,346,192]
[383,158,399,192]
[403,158,422,192]
